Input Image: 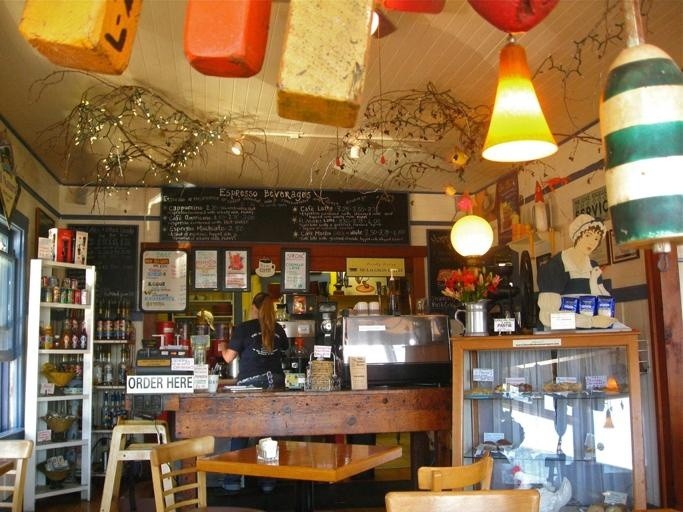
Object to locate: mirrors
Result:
[34,207,55,258]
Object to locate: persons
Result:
[537,213,629,329]
[222,292,289,493]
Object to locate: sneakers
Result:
[223,483,240,491]
[262,485,275,491]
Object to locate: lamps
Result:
[450,214,494,272]
[598,0,683,271]
[480,34,559,163]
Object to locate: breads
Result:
[587,502,629,512]
[463,384,534,394]
[543,381,583,392]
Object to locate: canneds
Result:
[41,276,89,305]
[60,362,83,379]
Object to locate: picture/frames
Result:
[610,229,640,264]
[222,247,251,292]
[589,231,610,266]
[191,246,222,292]
[279,247,310,294]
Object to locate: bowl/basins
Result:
[37,459,75,480]
[39,417,74,433]
[42,371,74,386]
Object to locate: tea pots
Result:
[274,304,289,322]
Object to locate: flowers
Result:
[440,264,504,303]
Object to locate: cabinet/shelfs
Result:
[170,292,235,328]
[91,338,135,477]
[22,259,97,512]
[447,325,647,512]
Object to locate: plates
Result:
[254,268,276,278]
[466,390,578,399]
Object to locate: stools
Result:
[100,418,177,512]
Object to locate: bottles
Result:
[333,283,343,296]
[277,337,307,391]
[95,292,133,476]
[40,318,86,348]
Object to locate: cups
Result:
[510,223,532,241]
[255,445,279,462]
[308,281,329,296]
[259,259,274,274]
[351,302,379,315]
[138,321,228,394]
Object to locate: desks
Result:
[196,440,402,511]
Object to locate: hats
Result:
[197,311,215,331]
[568,214,606,248]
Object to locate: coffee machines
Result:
[274,321,297,351]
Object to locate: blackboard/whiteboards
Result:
[65,223,139,310]
[159,186,411,244]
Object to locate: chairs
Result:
[417,451,493,491]
[0,439,33,512]
[383,489,541,512]
[149,435,215,512]
